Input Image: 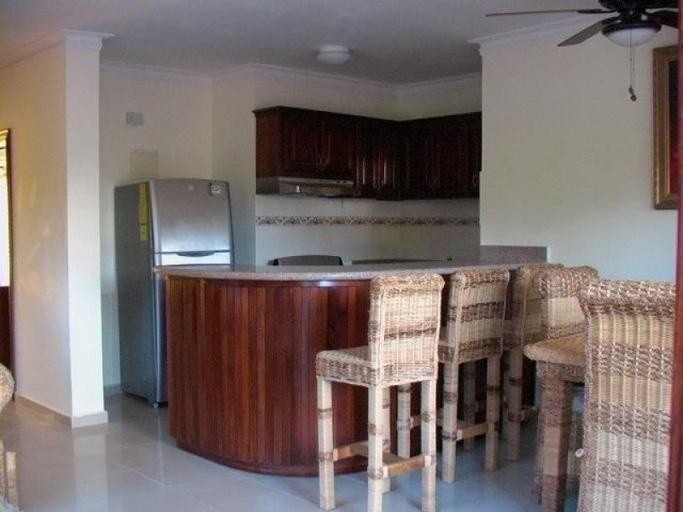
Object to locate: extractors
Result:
[255,176,354,199]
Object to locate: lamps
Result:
[602,16,660,48]
[316,43,351,64]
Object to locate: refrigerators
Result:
[111,176,236,409]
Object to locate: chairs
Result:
[317,274,446,511]
[395,265,510,484]
[532,267,600,512]
[576,279,673,511]
[461,262,562,463]
[273,255,343,266]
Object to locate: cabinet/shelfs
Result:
[252,105,481,200]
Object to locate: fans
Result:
[484,0,682,48]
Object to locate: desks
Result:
[521,331,582,511]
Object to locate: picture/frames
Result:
[652,44,682,210]
